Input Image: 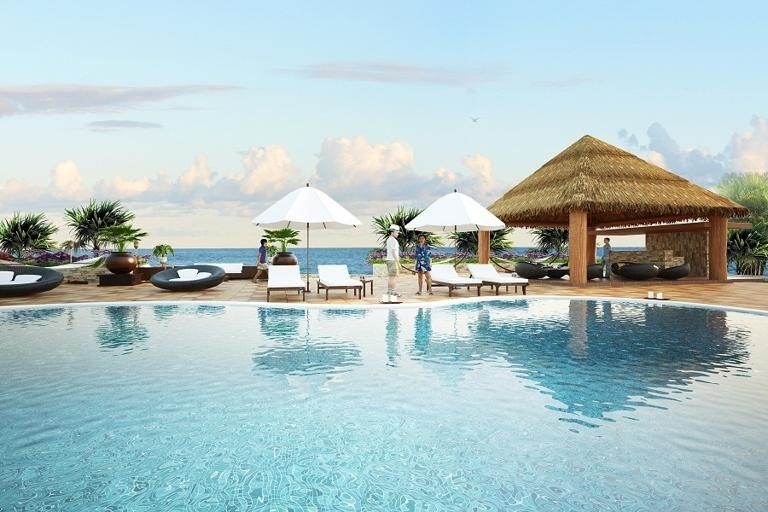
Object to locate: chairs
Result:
[266,264,305,301]
[423,264,483,297]
[465,263,529,296]
[316,264,362,301]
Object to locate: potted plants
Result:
[266,246,278,263]
[152,245,175,264]
[266,229,303,265]
[100,225,147,274]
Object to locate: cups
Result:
[657,292,662,299]
[648,291,654,297]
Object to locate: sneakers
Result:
[415,291,433,296]
[386,290,401,298]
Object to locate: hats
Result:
[387,223,400,231]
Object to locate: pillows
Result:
[0,270,14,282]
[196,272,212,279]
[177,269,198,278]
[14,275,43,282]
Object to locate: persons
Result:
[600,238,612,279]
[382,221,401,298]
[414,307,431,356]
[381,311,400,371]
[412,235,435,297]
[251,238,269,283]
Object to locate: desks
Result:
[361,279,374,297]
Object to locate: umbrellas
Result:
[250,309,367,391]
[403,188,505,290]
[255,183,362,294]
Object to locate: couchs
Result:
[0,264,64,298]
[516,259,695,281]
[150,265,226,291]
